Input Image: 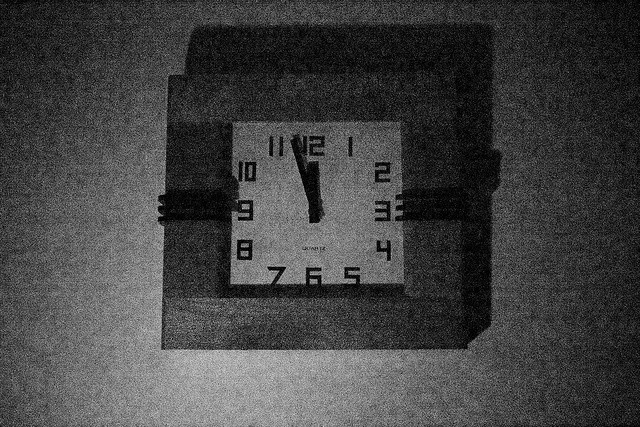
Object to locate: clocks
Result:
[159,26,493,350]
